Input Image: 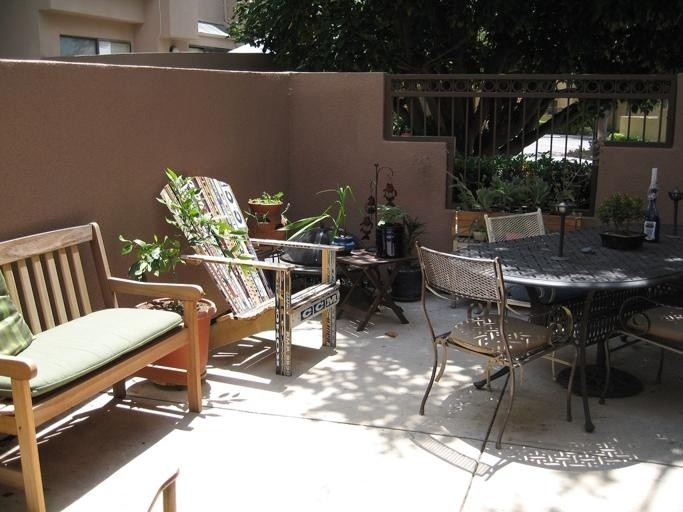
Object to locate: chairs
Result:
[158,174,344,379]
[413,240,582,450]
[597,292,681,416]
[483,209,549,238]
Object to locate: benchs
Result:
[0,221,206,510]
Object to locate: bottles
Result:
[643,188,660,243]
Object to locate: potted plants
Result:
[387,213,431,303]
[118,166,260,398]
[594,190,652,251]
[242,190,285,250]
[440,163,521,244]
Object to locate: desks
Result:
[335,250,420,331]
[444,225,682,424]
